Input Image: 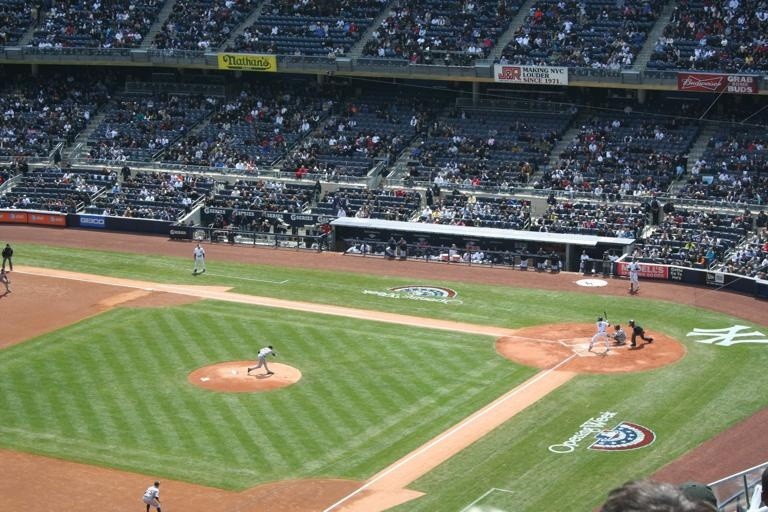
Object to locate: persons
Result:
[248,345,275,374]
[143,480,162,512]
[607,325,626,345]
[677,481,717,507]
[745,469,766,511]
[588,316,610,352]
[628,320,653,346]
[594,478,718,512]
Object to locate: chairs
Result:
[0,1,768,285]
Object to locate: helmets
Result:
[598,317,635,330]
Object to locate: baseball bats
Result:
[603,311,608,322]
